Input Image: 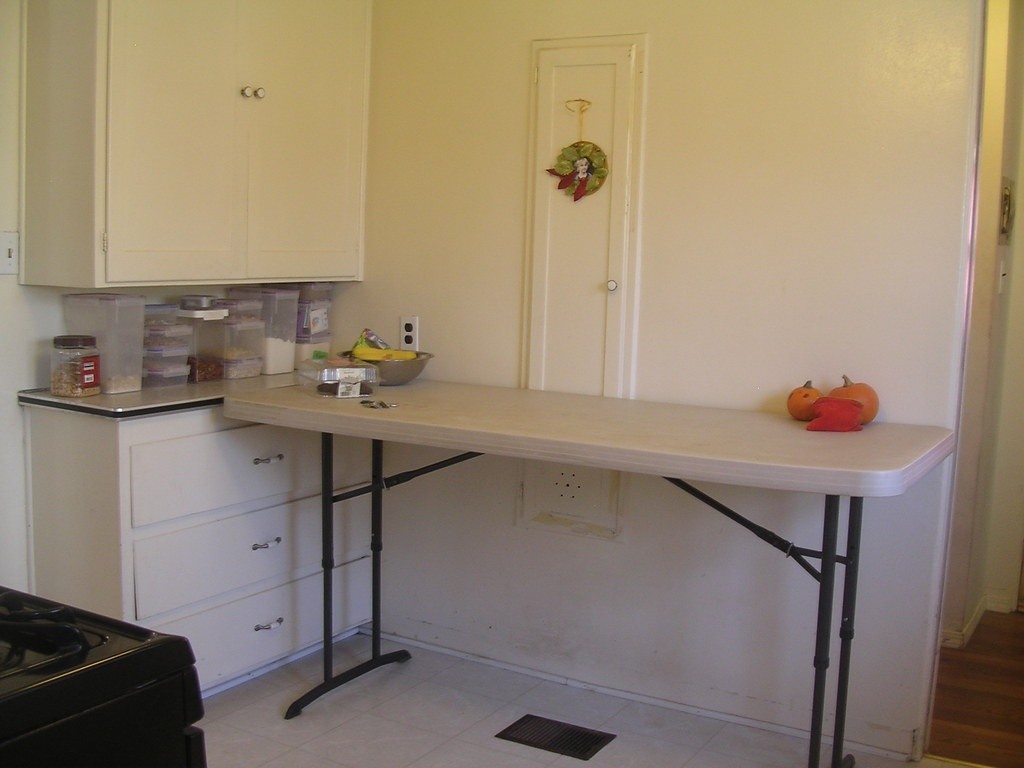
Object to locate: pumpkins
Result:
[788,381,824,420]
[829,375,879,424]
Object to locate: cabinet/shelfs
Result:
[23,405,374,700]
[22,0,373,288]
[530,45,632,525]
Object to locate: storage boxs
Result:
[62,282,332,394]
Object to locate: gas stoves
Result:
[0,586,207,768]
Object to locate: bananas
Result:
[352,338,417,360]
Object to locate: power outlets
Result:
[400,315,418,351]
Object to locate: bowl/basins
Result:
[335,350,435,385]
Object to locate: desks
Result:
[224,379,954,768]
